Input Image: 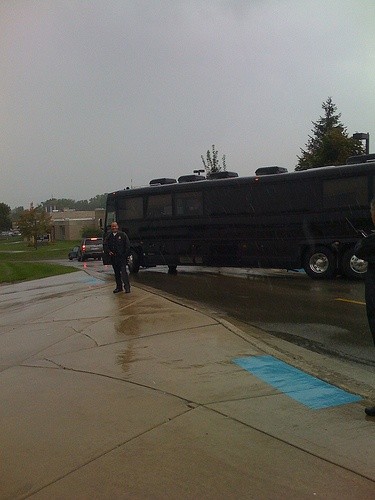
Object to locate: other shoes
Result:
[364,405,375,415]
[124,286,130,293]
[113,288,123,293]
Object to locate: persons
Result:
[352,200,375,416]
[103,220,130,293]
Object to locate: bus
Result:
[98,152,374,275]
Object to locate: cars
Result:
[67,246,80,260]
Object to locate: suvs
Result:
[77,237,104,262]
